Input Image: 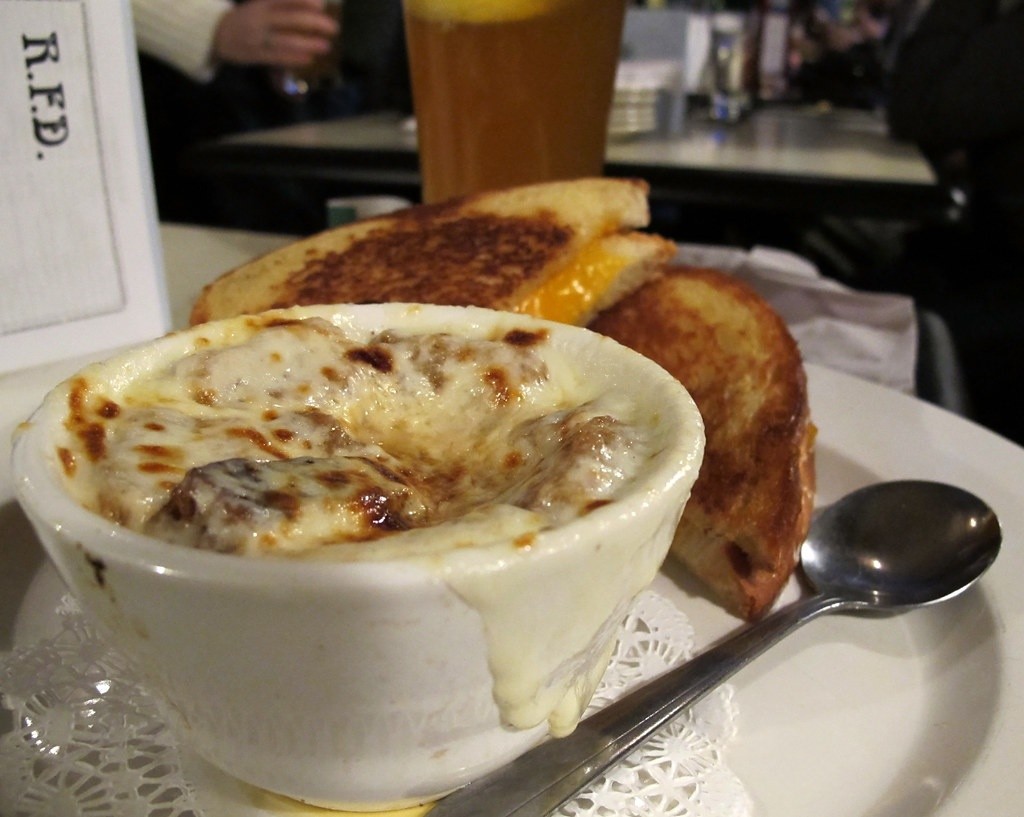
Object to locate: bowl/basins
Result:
[8,300,708,812]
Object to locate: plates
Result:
[12,359,1024,817]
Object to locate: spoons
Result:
[419,479,1005,817]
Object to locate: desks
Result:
[205,87,967,413]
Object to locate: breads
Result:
[195,178,814,621]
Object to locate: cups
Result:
[402,0,624,204]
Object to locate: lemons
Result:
[403,0,560,25]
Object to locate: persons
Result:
[781,0,1024,446]
[130,0,413,232]
